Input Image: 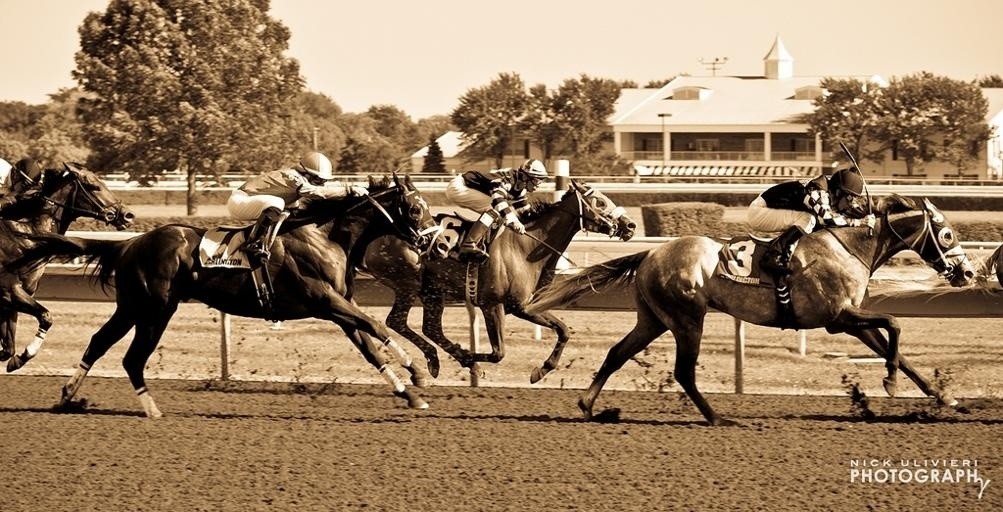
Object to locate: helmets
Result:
[298,152,333,180]
[831,169,863,196]
[522,159,549,178]
[14,159,41,186]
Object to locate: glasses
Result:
[845,194,853,203]
[519,167,541,187]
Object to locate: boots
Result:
[247,207,281,255]
[459,220,490,260]
[759,226,804,275]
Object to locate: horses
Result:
[1,160,1003,421]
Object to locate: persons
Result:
[745,167,877,279]
[445,158,548,260]
[0,156,42,194]
[226,151,334,260]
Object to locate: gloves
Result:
[347,186,370,198]
